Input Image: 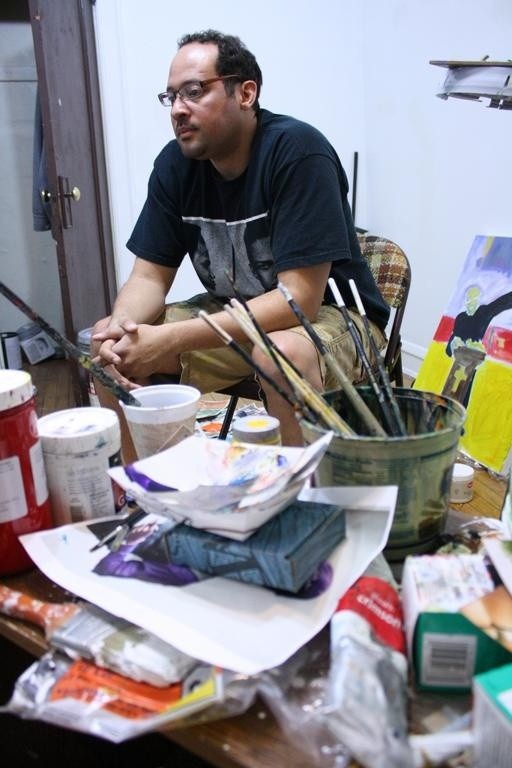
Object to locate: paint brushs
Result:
[0,282,141,407]
[90,507,144,553]
[197,276,409,437]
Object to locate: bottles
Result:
[232,415,282,446]
[36,406,128,532]
[0,369,54,579]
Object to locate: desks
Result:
[0,471,512,768]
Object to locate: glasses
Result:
[158,75,239,106]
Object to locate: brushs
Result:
[0,581,201,690]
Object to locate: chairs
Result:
[149,235,411,442]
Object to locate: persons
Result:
[445,284,512,436]
[90,28,386,464]
[182,222,216,290]
[243,218,278,292]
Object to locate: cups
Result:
[118,383,201,460]
